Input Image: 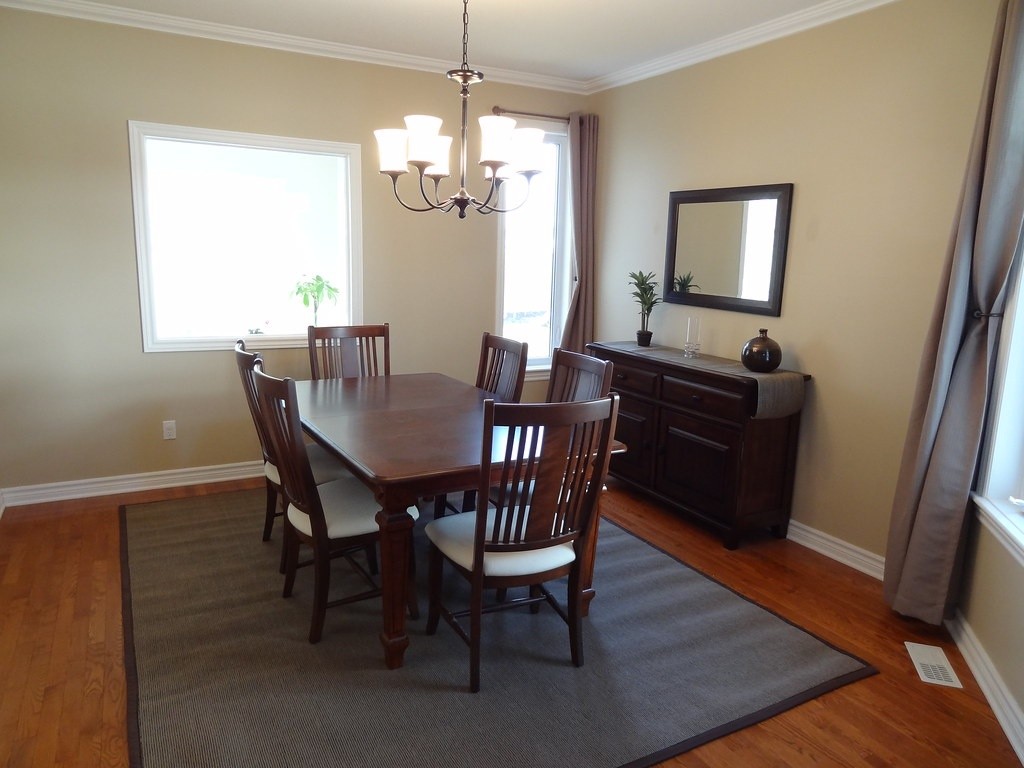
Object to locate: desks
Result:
[250,372,627,670]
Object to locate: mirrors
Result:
[662,180,795,318]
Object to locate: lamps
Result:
[376,0,545,217]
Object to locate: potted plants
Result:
[626,269,659,346]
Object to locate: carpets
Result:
[120,487,881,768]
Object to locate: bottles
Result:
[742,328,782,373]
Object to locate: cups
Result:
[684,317,702,358]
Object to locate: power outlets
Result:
[164,420,178,440]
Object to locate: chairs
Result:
[463,332,528,513]
[234,339,356,575]
[253,356,421,645]
[308,323,391,381]
[422,392,619,694]
[486,347,614,616]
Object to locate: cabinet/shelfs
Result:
[585,339,811,548]
